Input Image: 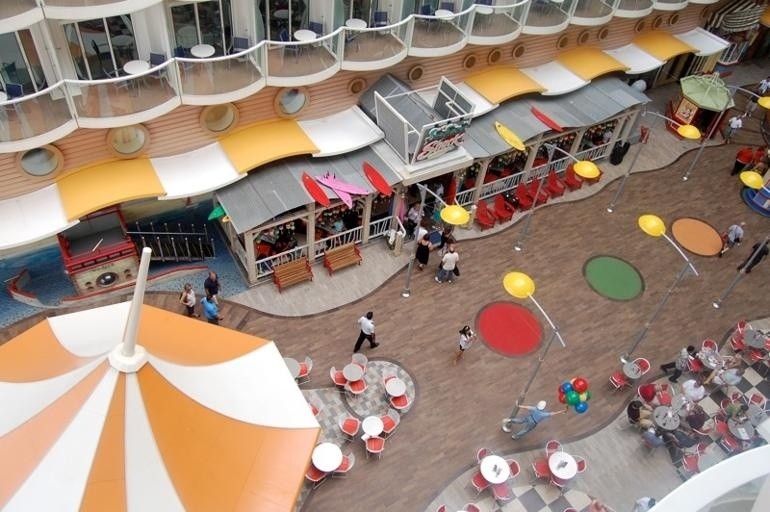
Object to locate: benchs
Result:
[323,241,362,276]
[272,256,314,293]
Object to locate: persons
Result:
[718,221,746,257]
[328,181,459,284]
[200,295,224,325]
[354,311,379,353]
[720,75,769,176]
[736,237,769,273]
[203,272,220,305]
[451,326,476,366]
[602,127,612,143]
[592,129,603,145]
[628,331,770,449]
[180,283,201,318]
[502,399,568,441]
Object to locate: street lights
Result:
[607,110,701,213]
[502,271,567,433]
[513,143,600,252]
[401,183,469,298]
[620,214,699,364]
[682,85,770,181]
[712,171,770,309]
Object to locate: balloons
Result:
[558,375,592,415]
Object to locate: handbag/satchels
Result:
[735,237,740,243]
[179,292,186,303]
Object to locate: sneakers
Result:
[501,418,520,440]
[718,248,725,258]
[192,314,200,318]
[434,276,442,284]
[452,353,465,366]
[370,341,379,349]
[418,264,422,270]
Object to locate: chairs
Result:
[419,2,458,33]
[92,38,167,101]
[171,35,253,83]
[531,1,562,18]
[0,59,29,117]
[610,321,770,478]
[476,159,604,232]
[344,11,390,53]
[437,440,608,512]
[278,22,324,67]
[284,353,413,491]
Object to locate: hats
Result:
[537,401,547,410]
[633,400,642,408]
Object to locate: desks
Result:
[472,6,494,27]
